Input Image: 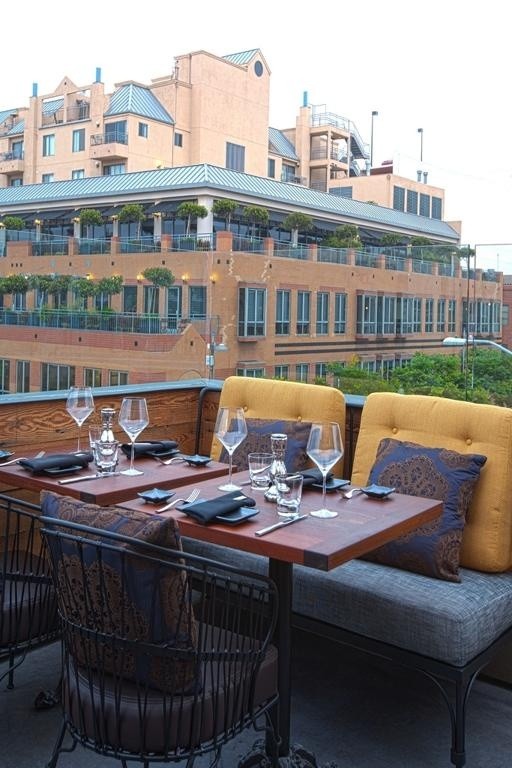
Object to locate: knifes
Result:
[57,471,123,485]
[254,513,309,537]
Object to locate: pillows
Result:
[38,488,206,698]
[219,416,321,477]
[364,437,486,584]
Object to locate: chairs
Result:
[2,494,74,709]
[38,515,320,766]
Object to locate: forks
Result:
[154,488,200,513]
[152,455,187,465]
[335,488,361,499]
[0,449,48,467]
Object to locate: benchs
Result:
[180,372,511,768]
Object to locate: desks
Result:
[117,465,447,764]
[1,442,237,507]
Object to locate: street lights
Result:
[443,333,511,357]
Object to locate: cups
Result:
[248,452,277,491]
[88,423,110,450]
[274,472,303,518]
[95,439,119,477]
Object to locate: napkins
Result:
[121,439,179,460]
[286,468,334,488]
[182,492,256,524]
[19,449,95,477]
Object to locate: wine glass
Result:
[214,406,248,492]
[117,396,150,476]
[306,420,345,519]
[66,385,95,456]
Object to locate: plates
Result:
[41,464,84,479]
[360,483,396,500]
[182,453,214,467]
[137,487,176,505]
[311,477,351,493]
[147,448,180,458]
[0,449,16,461]
[175,496,261,527]
